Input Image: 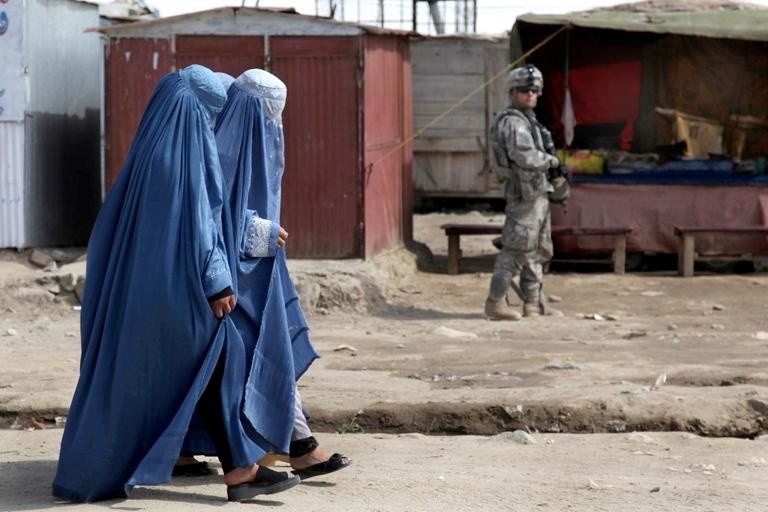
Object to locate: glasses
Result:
[521,85,537,92]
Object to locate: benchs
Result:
[440,223,633,276]
[672,224,767,276]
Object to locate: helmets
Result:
[505,67,543,91]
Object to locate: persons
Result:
[53,64,302,503]
[166,65,349,479]
[484,64,569,321]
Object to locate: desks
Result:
[537,158,767,270]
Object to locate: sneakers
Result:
[523,301,563,318]
[484,298,521,320]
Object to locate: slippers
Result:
[289,453,351,480]
[171,461,217,476]
[227,466,300,501]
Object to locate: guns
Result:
[543,127,570,212]
